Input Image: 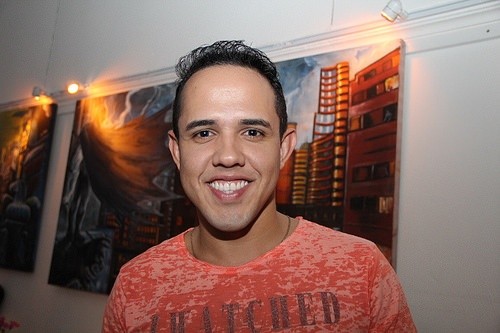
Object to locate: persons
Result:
[102,39,419,332]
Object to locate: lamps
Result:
[32,85,46,98]
[381,0,408,22]
[66,80,84,94]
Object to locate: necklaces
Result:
[190,213,291,260]
